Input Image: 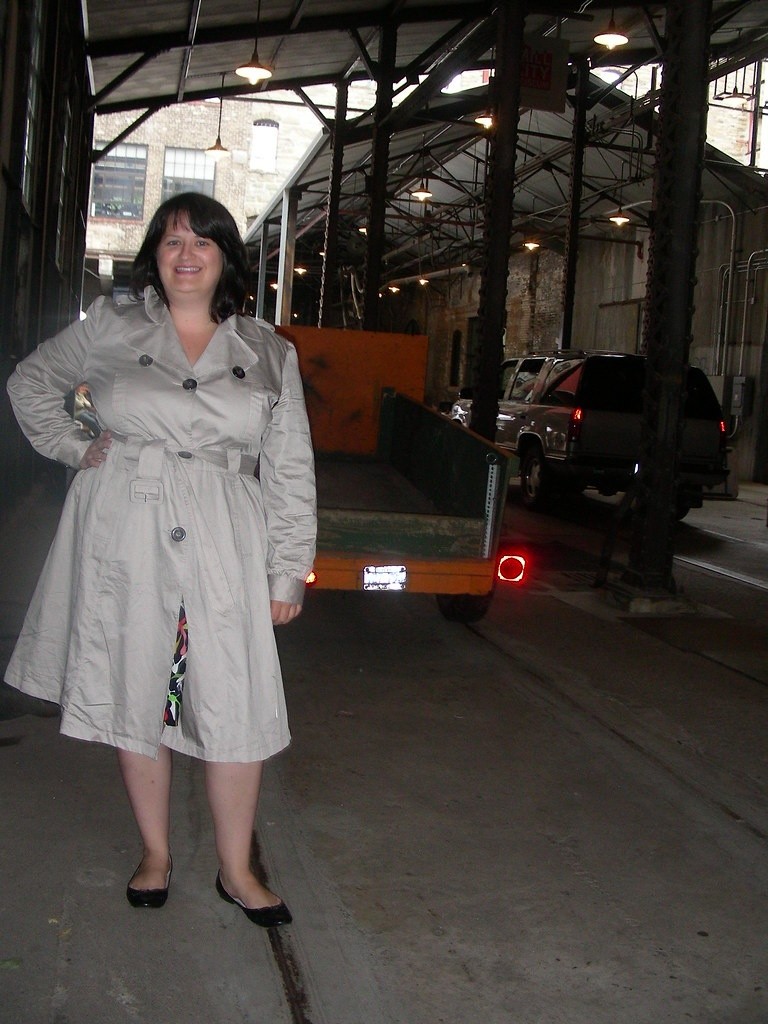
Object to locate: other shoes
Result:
[126,853,173,909]
[215,868,294,928]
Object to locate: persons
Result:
[7,193,319,934]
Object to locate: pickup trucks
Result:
[449,350,742,516]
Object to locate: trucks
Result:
[267,328,516,627]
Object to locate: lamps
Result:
[235,0,272,85]
[204,74,228,158]
[593,9,630,50]
[721,70,747,106]
[609,160,629,226]
[411,46,497,201]
[522,197,539,249]
